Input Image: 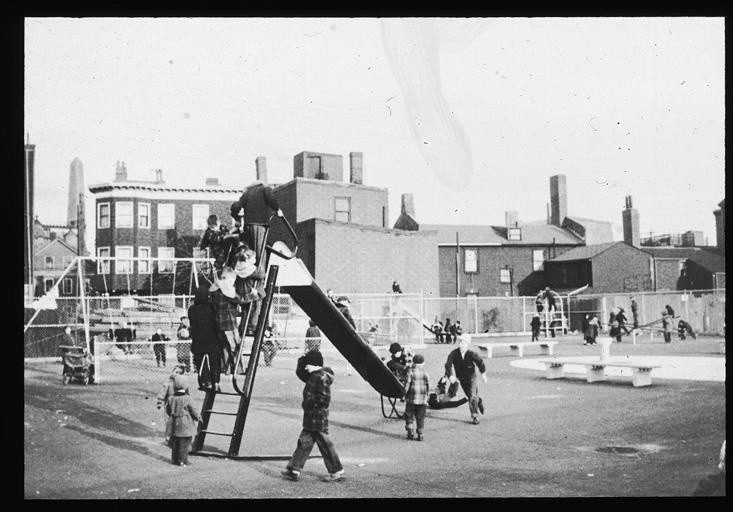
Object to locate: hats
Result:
[413,355,425,363]
[173,375,189,393]
[304,350,323,366]
[337,296,351,303]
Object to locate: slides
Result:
[278,255,405,396]
[402,305,455,336]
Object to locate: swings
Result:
[101,261,135,349]
[149,260,179,349]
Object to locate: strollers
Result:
[57,344,94,385]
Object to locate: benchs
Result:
[538,353,660,390]
[470,338,556,360]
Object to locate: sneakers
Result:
[479,398,484,415]
[472,417,480,424]
[171,458,193,466]
[281,468,300,481]
[407,430,424,441]
[321,469,346,482]
